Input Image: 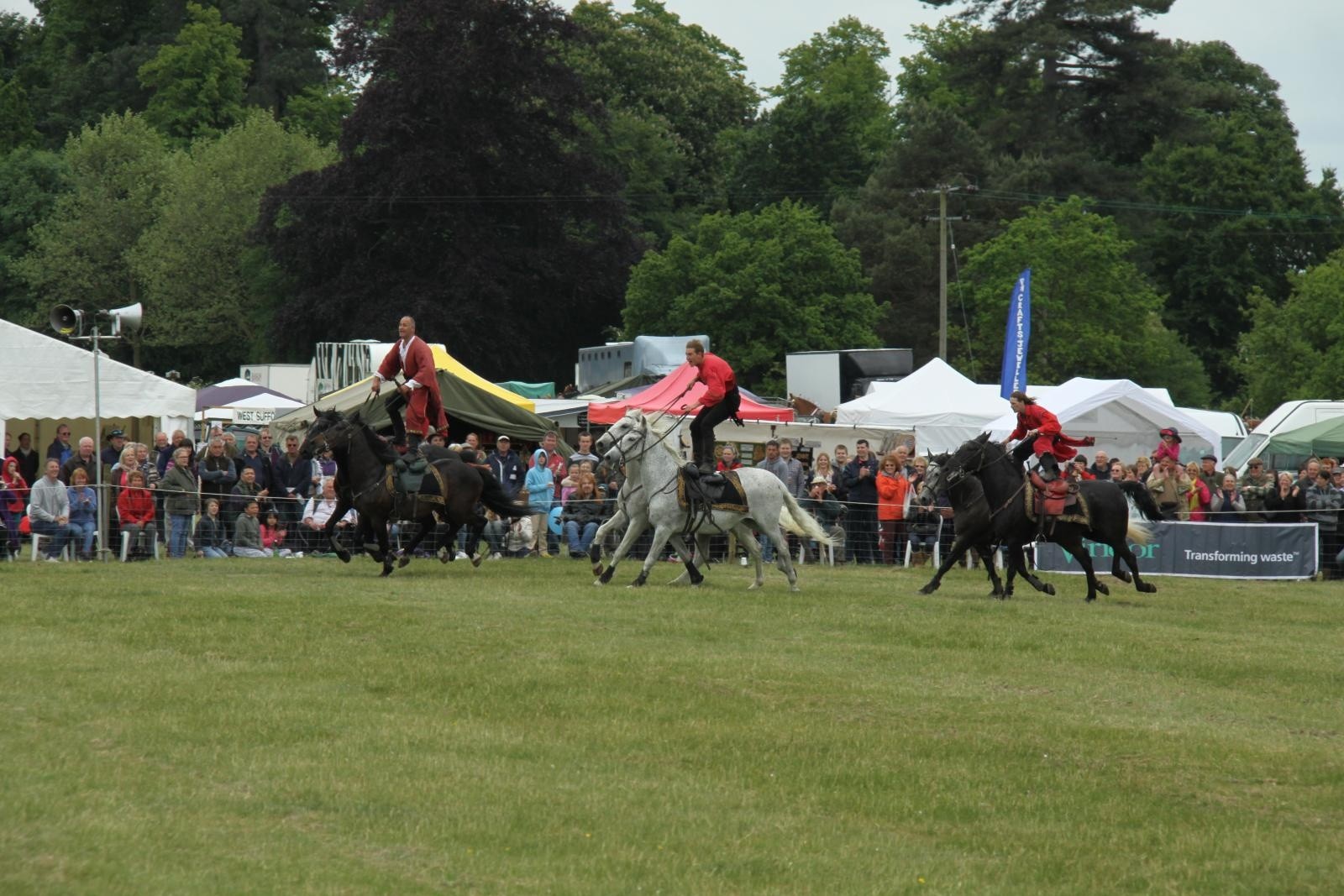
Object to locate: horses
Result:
[603,411,846,594]
[590,404,834,586]
[307,409,553,579]
[298,405,485,563]
[917,430,1174,603]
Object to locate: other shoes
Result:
[2,536,939,567]
[1041,470,1061,480]
[387,437,407,447]
[409,440,419,453]
[698,463,714,474]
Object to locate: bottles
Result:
[826,479,832,490]
[296,493,305,505]
[273,540,280,557]
[555,470,561,484]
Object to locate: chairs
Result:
[27,504,101,562]
[904,514,943,570]
[798,523,838,566]
[116,506,159,562]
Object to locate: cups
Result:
[549,482,554,488]
[740,557,748,565]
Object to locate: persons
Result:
[1063,427,1344,583]
[0,424,693,562]
[372,316,447,463]
[1000,391,1095,481]
[713,438,957,565]
[681,339,740,474]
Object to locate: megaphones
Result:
[109,302,142,332]
[50,305,85,334]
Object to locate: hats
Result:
[1160,427,1182,444]
[1200,455,1217,462]
[1332,468,1344,474]
[1247,458,1263,465]
[498,436,509,441]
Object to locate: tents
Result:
[269,346,577,461]
[834,357,1225,472]
[0,320,197,562]
[588,361,794,461]
[196,377,308,447]
[1268,417,1344,470]
